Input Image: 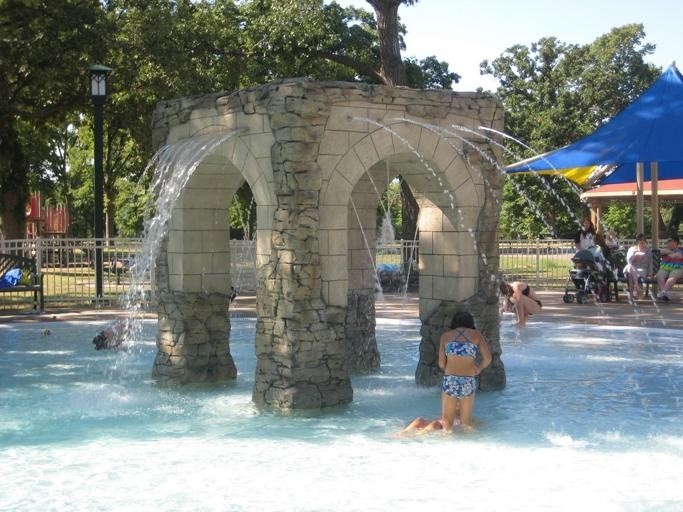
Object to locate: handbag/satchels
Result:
[0,268,33,287]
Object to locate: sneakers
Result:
[627,289,644,305]
[657,290,670,301]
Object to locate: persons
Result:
[574,218,597,254]
[654,236,683,301]
[622,233,654,304]
[91,319,137,350]
[437,309,493,432]
[403,401,462,436]
[499,280,543,328]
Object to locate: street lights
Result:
[85,58,115,302]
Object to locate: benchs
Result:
[605,248,682,302]
[0,254,45,313]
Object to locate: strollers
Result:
[562,241,616,304]
